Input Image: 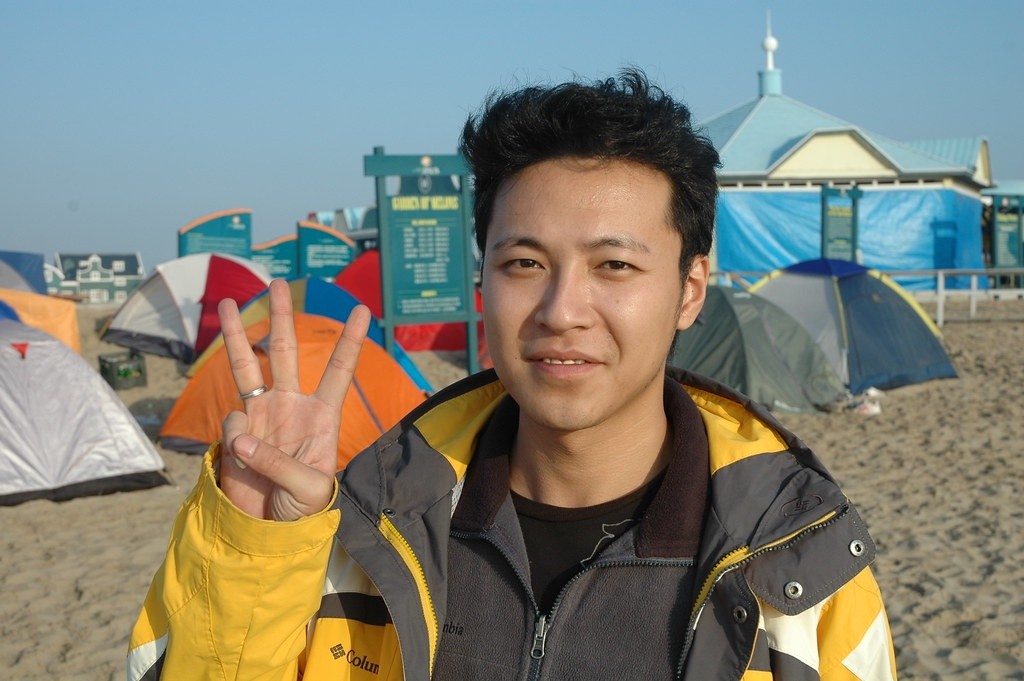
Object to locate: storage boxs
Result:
[99,352,147,390]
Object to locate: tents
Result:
[98,253,274,365]
[181,275,437,394]
[0,250,80,350]
[0,317,177,505]
[156,313,427,474]
[748,257,958,396]
[335,250,484,351]
[670,284,846,414]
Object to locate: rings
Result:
[239,385,267,400]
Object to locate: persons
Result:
[127,66,897,681]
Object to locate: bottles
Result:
[118,364,132,377]
